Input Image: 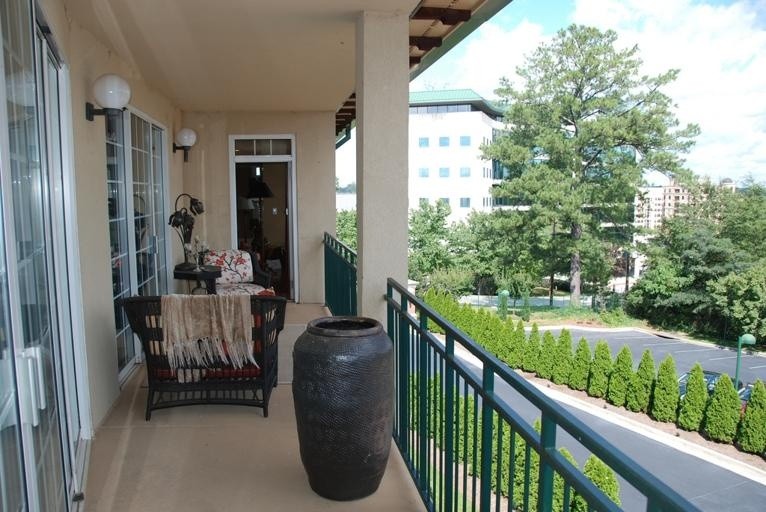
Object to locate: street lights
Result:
[495,288,511,317]
[735,332,758,400]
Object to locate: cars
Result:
[668,365,766,428]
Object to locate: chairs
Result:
[197,249,265,296]
[121,293,288,421]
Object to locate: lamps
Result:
[173,127,197,161]
[247,181,274,224]
[85,74,131,132]
[168,193,204,271]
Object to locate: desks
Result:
[175,264,222,295]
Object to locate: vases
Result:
[291,315,397,501]
